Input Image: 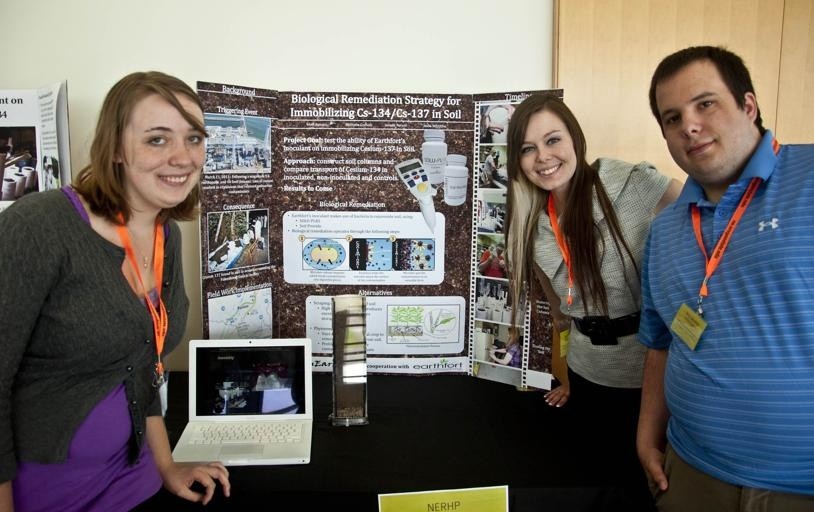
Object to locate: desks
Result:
[128,370,657,511]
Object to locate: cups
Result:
[1,165,35,200]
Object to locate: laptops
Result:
[171,338,313,467]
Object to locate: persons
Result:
[493,89,686,473]
[481,149,498,187]
[476,241,511,279]
[44,164,56,191]
[487,326,523,373]
[0,68,238,512]
[634,44,813,512]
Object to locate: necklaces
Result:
[128,223,155,270]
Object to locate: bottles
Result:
[443,154,468,206]
[420,129,447,185]
